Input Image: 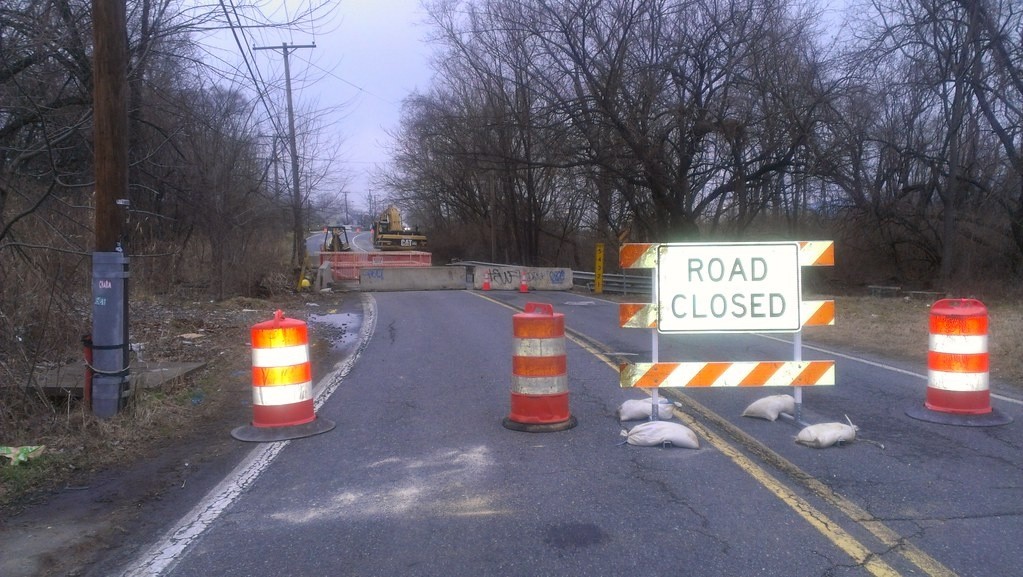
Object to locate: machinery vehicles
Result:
[320,224,351,253]
[369,206,428,252]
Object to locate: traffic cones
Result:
[482,270,492,291]
[517,270,531,294]
